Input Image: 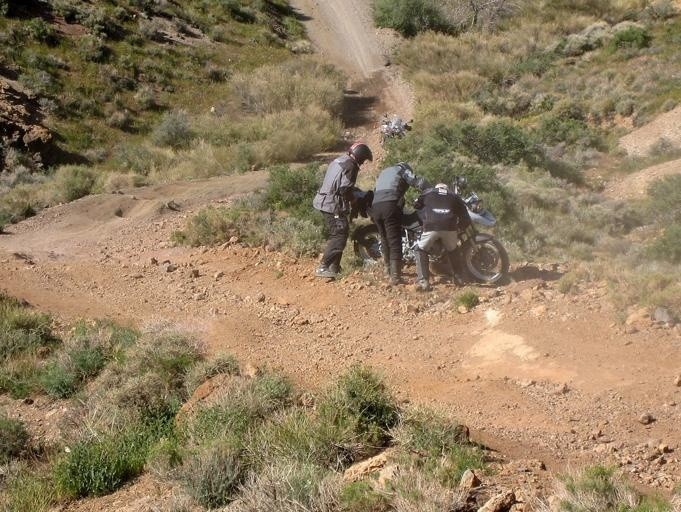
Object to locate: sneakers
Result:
[329,262,341,274]
[314,264,337,277]
[416,277,430,290]
[451,277,464,286]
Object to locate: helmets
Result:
[435,183,449,191]
[396,162,413,175]
[348,143,372,163]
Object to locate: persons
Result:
[313,143,373,277]
[414,183,472,290]
[370,162,434,283]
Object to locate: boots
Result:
[389,260,407,285]
[384,257,390,274]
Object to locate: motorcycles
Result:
[350,176,511,286]
[379,112,414,148]
